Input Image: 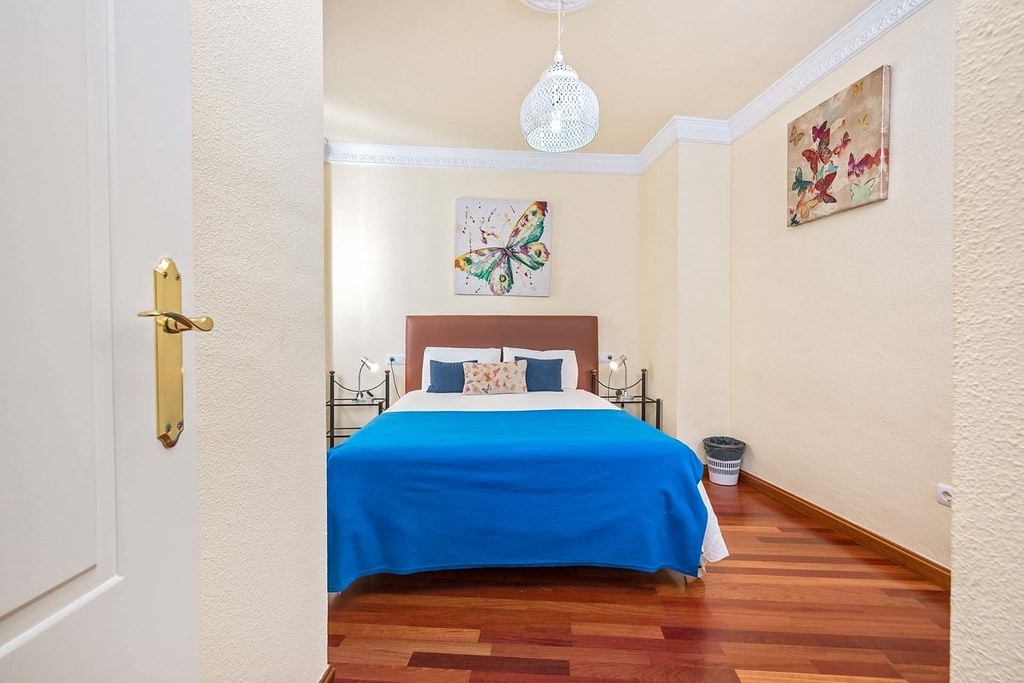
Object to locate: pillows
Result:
[422,346,502,392]
[514,356,564,392]
[426,359,478,393]
[503,346,579,390]
[461,359,528,395]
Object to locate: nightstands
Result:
[591,368,661,430]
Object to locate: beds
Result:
[327,314,730,593]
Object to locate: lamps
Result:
[355,356,380,401]
[520,0,600,153]
[609,354,635,400]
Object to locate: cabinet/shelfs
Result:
[326,370,390,449]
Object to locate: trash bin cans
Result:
[703,436,746,486]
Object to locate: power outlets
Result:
[386,354,404,365]
[936,483,951,508]
[598,352,614,362]
